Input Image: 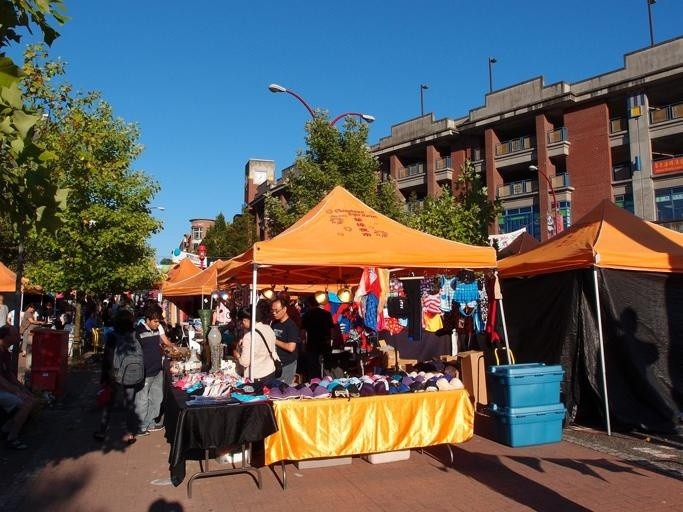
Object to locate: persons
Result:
[105,316,136,445]
[20,301,45,357]
[257,299,272,325]
[85,311,102,347]
[269,298,301,385]
[299,293,336,379]
[59,314,75,359]
[275,289,305,343]
[0,295,9,329]
[0,324,39,451]
[86,296,96,321]
[7,304,26,328]
[135,306,180,437]
[232,304,283,383]
[101,292,158,348]
[92,308,132,442]
[42,304,49,323]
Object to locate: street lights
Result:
[528,164,563,238]
[267,82,375,127]
[196,244,207,270]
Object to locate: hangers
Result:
[399,271,424,281]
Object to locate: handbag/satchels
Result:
[96,384,113,408]
[275,360,282,378]
[19,324,26,335]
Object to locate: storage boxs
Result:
[290,456,353,468]
[358,450,411,464]
[453,350,488,406]
[494,407,567,448]
[492,362,565,407]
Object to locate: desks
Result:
[264,389,475,490]
[163,363,279,498]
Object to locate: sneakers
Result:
[147,424,165,431]
[5,439,30,450]
[93,432,105,441]
[135,427,150,436]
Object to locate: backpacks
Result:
[108,337,145,386]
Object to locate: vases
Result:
[207,326,221,347]
[199,310,212,339]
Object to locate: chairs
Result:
[92,328,104,353]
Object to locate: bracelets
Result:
[235,355,241,360]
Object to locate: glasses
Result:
[270,309,283,313]
